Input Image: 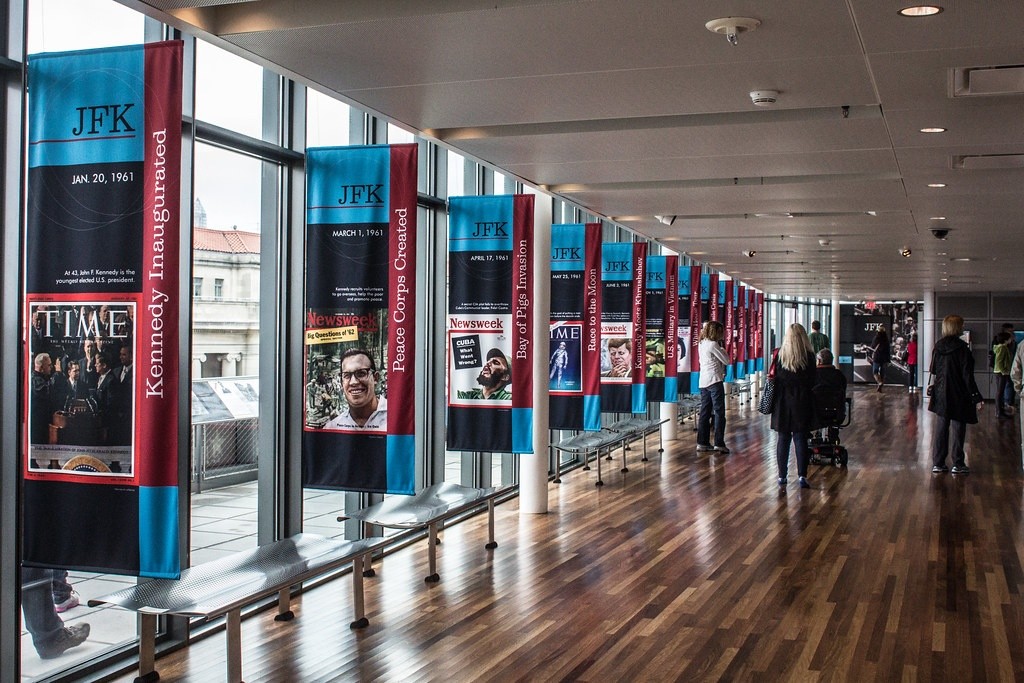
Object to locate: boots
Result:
[873,373,883,393]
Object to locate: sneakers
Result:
[696,443,714,451]
[714,446,730,453]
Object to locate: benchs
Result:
[549,427,636,487]
[601,417,671,462]
[730,382,755,406]
[678,398,702,432]
[338,483,511,584]
[87,531,374,682]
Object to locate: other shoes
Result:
[994,412,1014,420]
[799,476,811,489]
[951,465,970,472]
[777,478,787,485]
[913,386,920,389]
[1005,403,1019,412]
[933,465,949,472]
[909,386,912,389]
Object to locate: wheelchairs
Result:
[805,384,851,467]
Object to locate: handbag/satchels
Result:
[756,349,781,415]
[988,350,995,369]
[926,349,935,396]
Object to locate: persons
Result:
[601,338,632,377]
[870,325,891,391]
[457,348,512,400]
[21,567,90,660]
[770,323,820,489]
[323,347,387,432]
[905,317,916,336]
[696,320,729,453]
[807,349,847,444]
[677,336,687,372]
[874,304,886,315]
[1010,339,1024,471]
[929,314,985,472]
[907,333,922,389]
[807,321,830,354]
[991,324,1018,418]
[31,305,132,447]
[771,329,775,354]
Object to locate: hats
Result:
[608,338,631,354]
[487,348,512,383]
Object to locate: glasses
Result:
[339,367,375,382]
